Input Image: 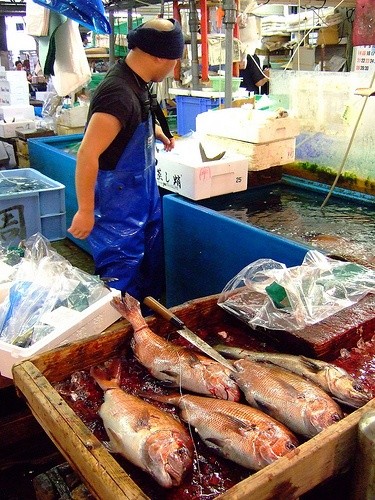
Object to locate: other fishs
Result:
[91,294,370,491]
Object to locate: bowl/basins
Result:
[209,76,244,93]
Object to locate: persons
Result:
[80,32,90,48]
[14,61,24,71]
[67,17,185,317]
[240,54,270,95]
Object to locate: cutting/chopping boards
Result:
[224,291,375,361]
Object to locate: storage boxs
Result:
[0,65,375,378]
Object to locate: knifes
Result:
[143,295,238,373]
